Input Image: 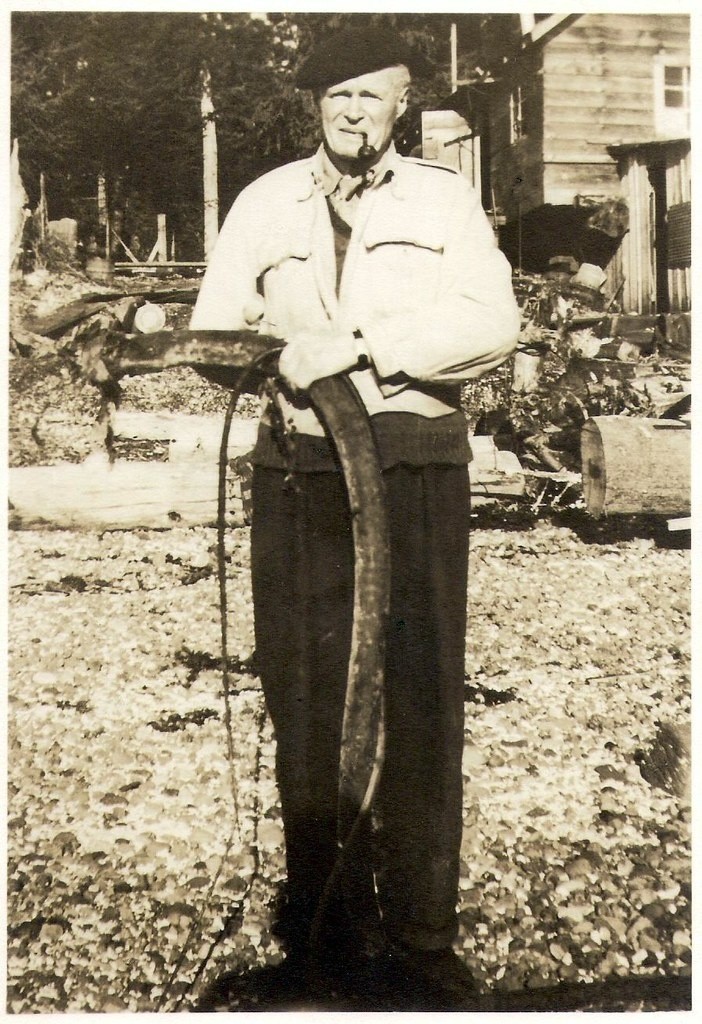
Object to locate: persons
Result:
[190,30,526,1012]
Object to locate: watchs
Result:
[353,330,369,370]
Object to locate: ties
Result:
[334,178,361,229]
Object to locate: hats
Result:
[295,26,434,90]
